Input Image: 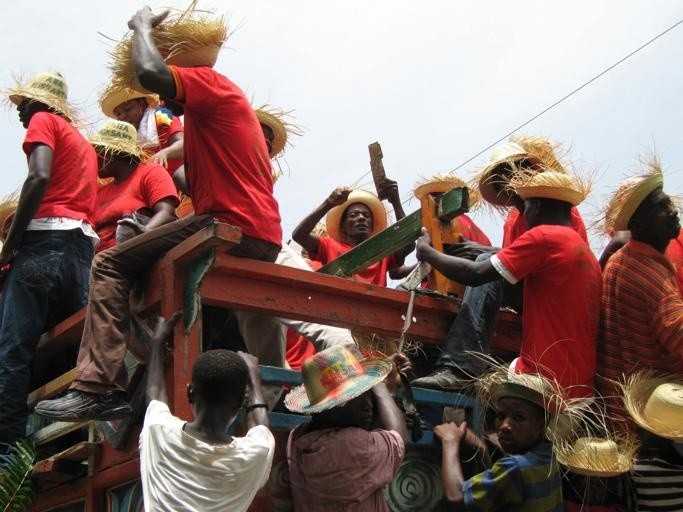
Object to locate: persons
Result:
[34,6,280,421]
[2,75,680,512]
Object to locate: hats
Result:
[488,373,683,478]
[590,147,663,239]
[0,197,17,233]
[8,18,287,160]
[477,137,592,216]
[283,344,393,415]
[408,173,485,208]
[325,189,387,242]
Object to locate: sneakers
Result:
[35,386,132,422]
[411,367,479,395]
